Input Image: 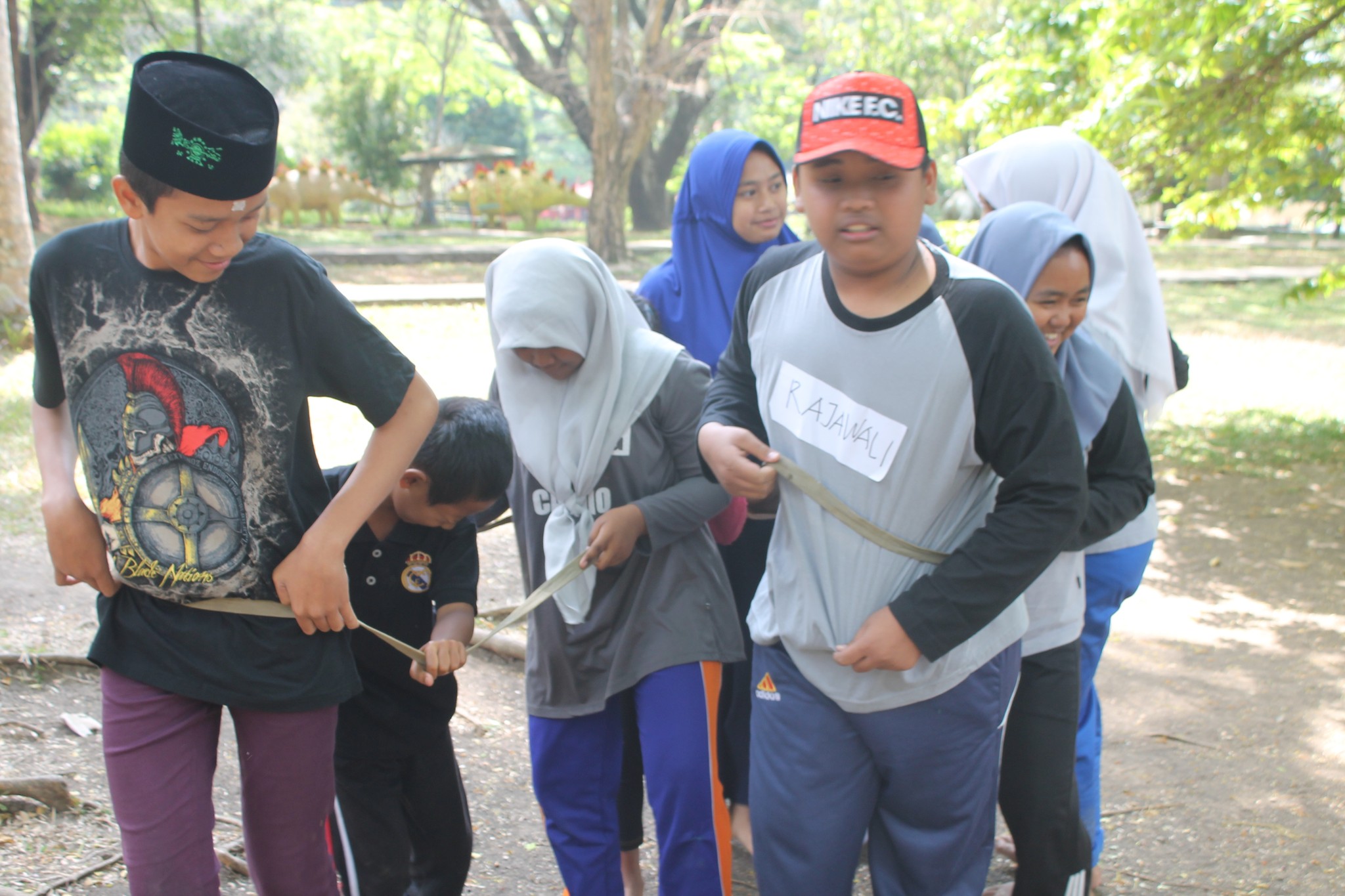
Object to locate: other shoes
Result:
[731,833,759,896]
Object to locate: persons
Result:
[484,237,738,896]
[693,74,1085,888]
[952,125,1191,896]
[318,395,512,896]
[623,128,801,856]
[27,50,439,896]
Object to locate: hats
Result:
[793,71,929,170]
[123,51,279,200]
